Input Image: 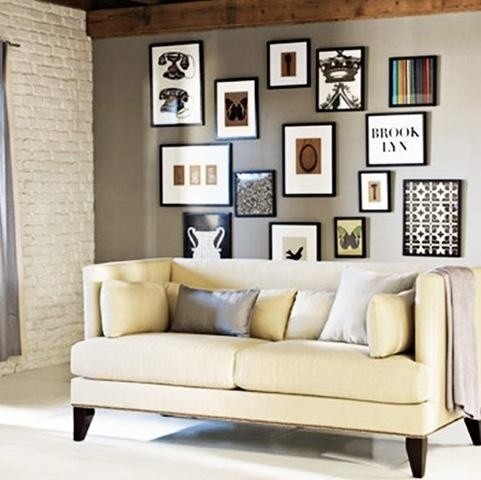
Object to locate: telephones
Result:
[159,88,188,113]
[158,52,189,80]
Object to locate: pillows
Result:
[319,262,420,347]
[163,280,297,341]
[287,286,337,344]
[362,287,419,361]
[172,281,261,337]
[95,276,171,342]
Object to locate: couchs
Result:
[68,258,481,479]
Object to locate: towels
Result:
[430,262,480,423]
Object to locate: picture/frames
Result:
[234,168,278,219]
[212,77,263,142]
[357,169,394,214]
[315,44,368,114]
[332,214,369,259]
[267,220,323,261]
[402,175,465,258]
[180,210,233,260]
[365,111,431,168]
[388,53,442,109]
[281,119,339,199]
[265,36,314,91]
[157,139,234,208]
[148,38,207,129]
